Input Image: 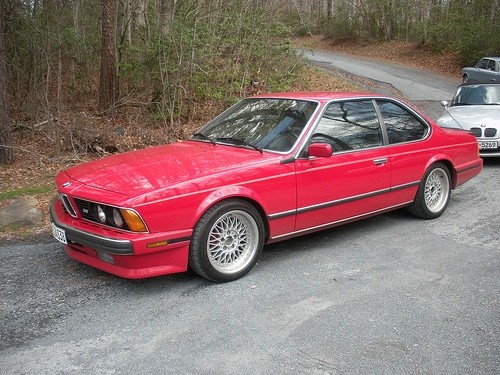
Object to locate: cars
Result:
[462,57,500,85]
[49,91,483,282]
[435,84,500,157]
